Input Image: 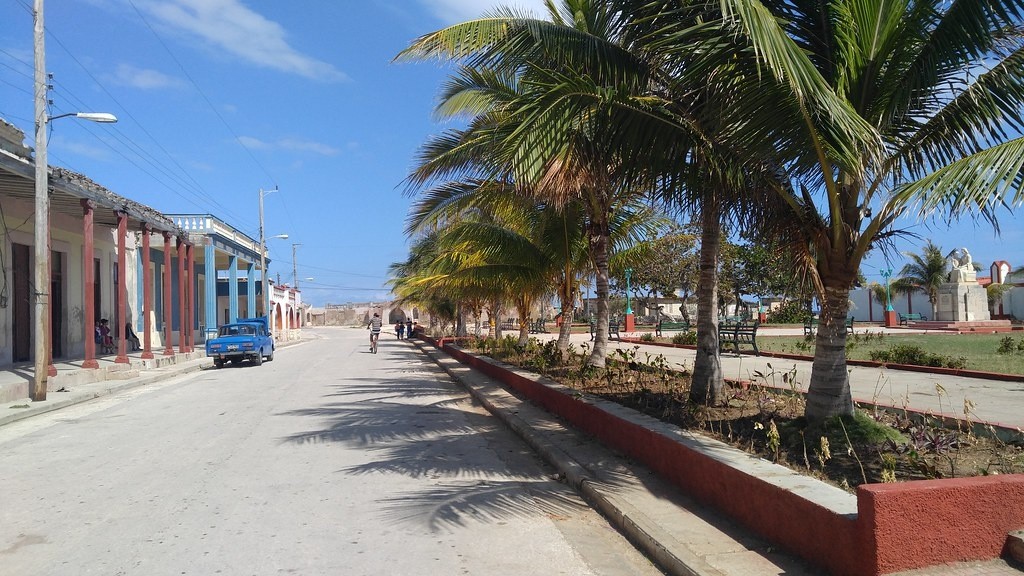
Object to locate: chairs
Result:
[229,329,239,335]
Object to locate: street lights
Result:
[33,110,119,402]
[621,266,637,332]
[257,232,289,338]
[880,267,897,328]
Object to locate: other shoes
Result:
[132,348,136,351]
[137,348,143,351]
[369,347,373,350]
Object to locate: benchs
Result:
[804,315,854,336]
[655,319,690,337]
[718,318,760,357]
[898,312,928,326]
[528,318,546,334]
[501,318,515,330]
[590,320,622,342]
[482,321,491,329]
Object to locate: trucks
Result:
[205,316,276,368]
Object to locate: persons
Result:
[125,323,140,351]
[367,312,382,350]
[95,319,115,354]
[395,318,422,340]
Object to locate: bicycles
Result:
[367,326,380,354]
